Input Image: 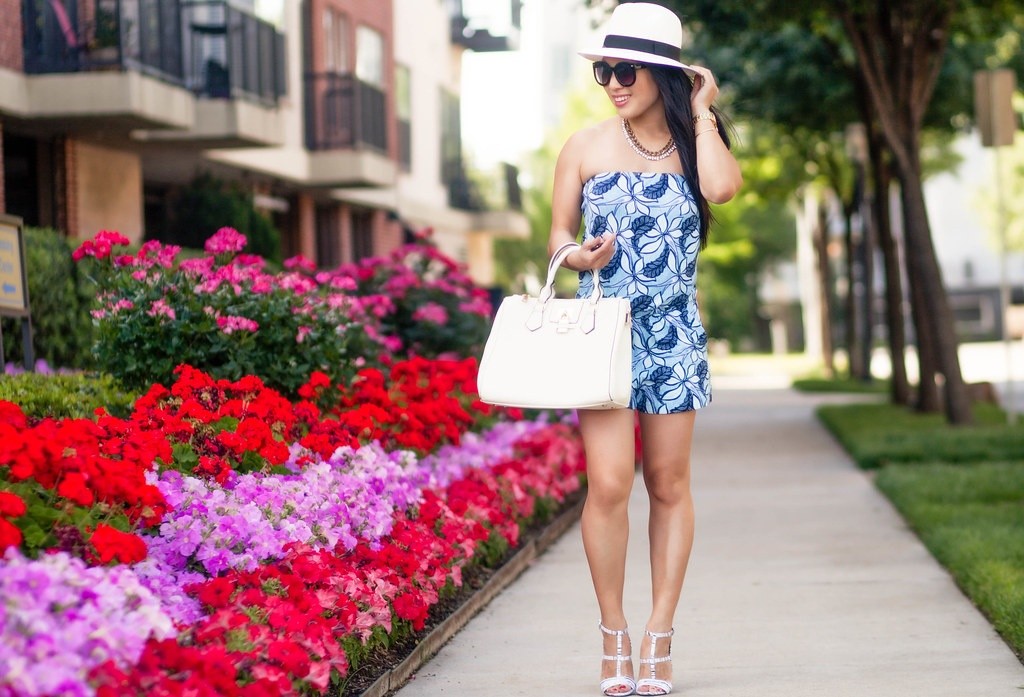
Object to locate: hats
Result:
[578,2,691,68]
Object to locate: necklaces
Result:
[622,118,676,160]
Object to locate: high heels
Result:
[599,620,635,697]
[635,627,674,695]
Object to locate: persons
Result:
[546,2,742,697]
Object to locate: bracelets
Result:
[691,112,717,128]
[695,128,719,138]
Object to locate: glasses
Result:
[592,60,649,87]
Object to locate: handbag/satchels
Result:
[476,243,632,409]
